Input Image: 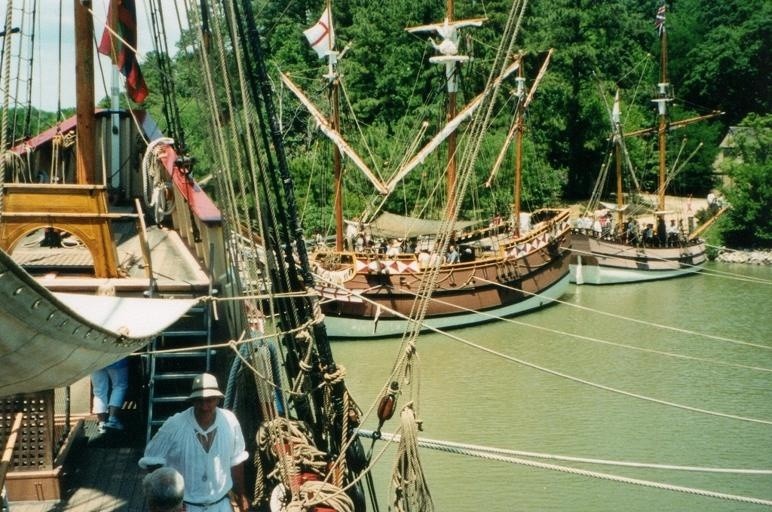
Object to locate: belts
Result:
[183,493,228,508]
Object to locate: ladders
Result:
[146,243,215,448]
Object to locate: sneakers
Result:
[96,419,126,435]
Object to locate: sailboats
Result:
[286,0,575,336]
[1,0,379,511]
[567,4,732,285]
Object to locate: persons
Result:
[716,192,724,212]
[89,354,135,437]
[312,204,524,264]
[137,370,253,511]
[685,193,695,215]
[140,466,188,511]
[575,211,684,250]
[706,189,715,207]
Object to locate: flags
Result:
[301,2,337,61]
[653,3,666,39]
[95,0,150,103]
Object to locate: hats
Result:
[185,372,227,403]
